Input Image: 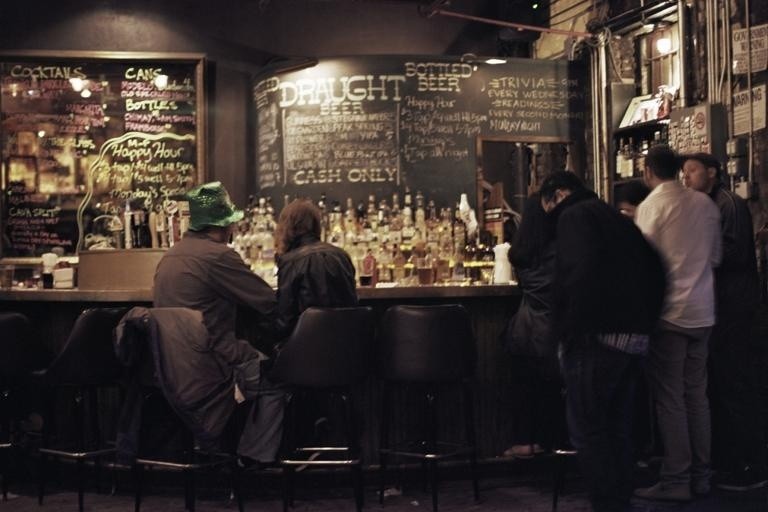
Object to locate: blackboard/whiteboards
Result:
[245,52,587,213]
[0,49,205,257]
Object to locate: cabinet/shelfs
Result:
[608,81,670,208]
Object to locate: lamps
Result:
[417,1,604,39]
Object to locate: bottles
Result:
[231,190,495,284]
[616,129,671,178]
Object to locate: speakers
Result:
[498,0,536,43]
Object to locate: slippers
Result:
[504,444,544,459]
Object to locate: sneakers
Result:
[235,416,334,470]
[634,460,767,501]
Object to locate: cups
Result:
[417,255,434,286]
[493,244,512,285]
[356,257,374,287]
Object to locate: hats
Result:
[680,152,722,172]
[187,181,244,232]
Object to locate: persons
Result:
[630,144,726,504]
[152,180,290,478]
[535,169,668,509]
[501,191,571,462]
[677,152,768,495]
[270,197,358,323]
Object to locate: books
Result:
[119,209,190,251]
